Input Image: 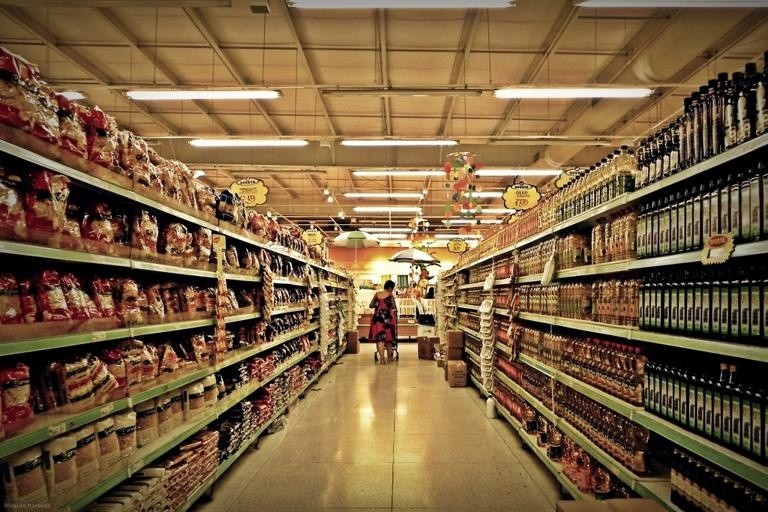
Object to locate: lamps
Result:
[493,8,655,99]
[337,139,482,240]
[40,6,284,100]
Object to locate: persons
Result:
[370,280,397,364]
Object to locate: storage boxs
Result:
[347,330,361,354]
[416,337,445,367]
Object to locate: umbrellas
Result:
[332,230,380,263]
[389,247,440,282]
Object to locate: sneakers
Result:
[381,356,395,364]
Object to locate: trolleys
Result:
[372,311,402,361]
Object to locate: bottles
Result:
[456,49,768,512]
